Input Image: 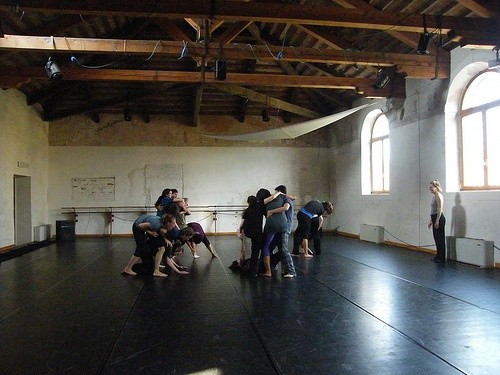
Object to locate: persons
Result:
[242,185,296,278]
[291,199,334,258]
[428,180,446,263]
[123,189,217,277]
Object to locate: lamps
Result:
[234,95,249,123]
[216,56,228,80]
[415,15,433,55]
[124,108,131,122]
[262,109,271,122]
[44,55,64,80]
[372,69,391,90]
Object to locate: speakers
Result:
[56,220,75,242]
